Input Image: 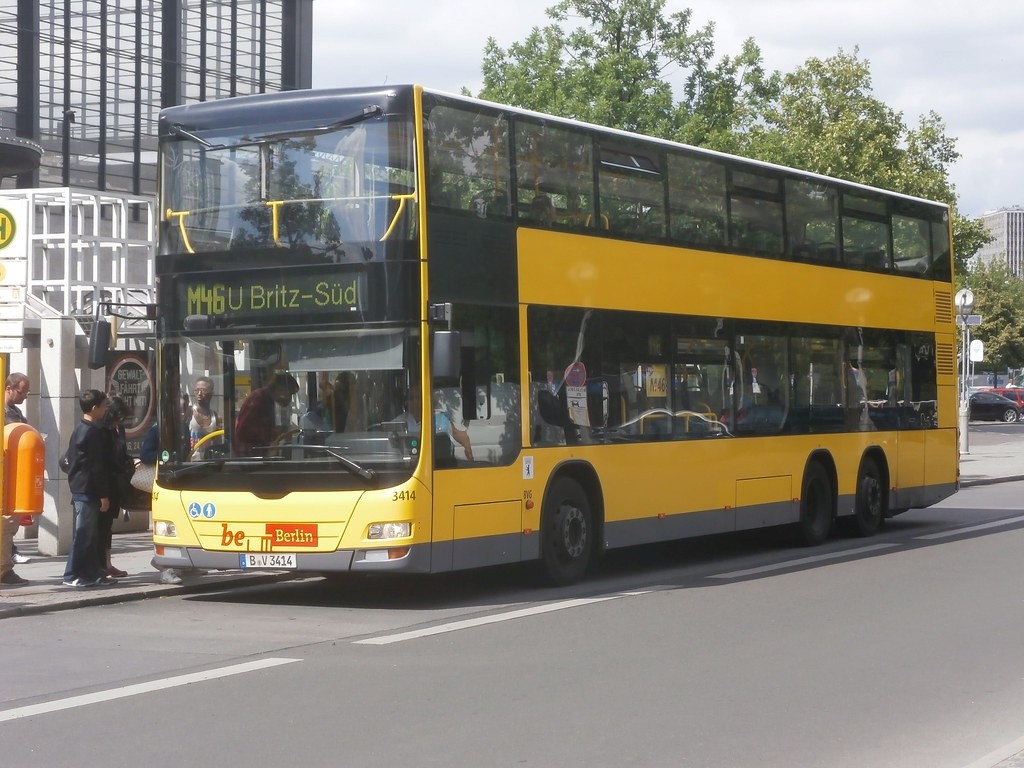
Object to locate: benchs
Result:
[778,405,914,434]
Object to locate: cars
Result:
[978,388,1024,422]
[968,392,1020,422]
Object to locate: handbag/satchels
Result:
[130,462,157,494]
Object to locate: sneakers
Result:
[161,568,182,584]
[105,565,128,577]
[62,576,94,588]
[0,571,29,586]
[11,552,31,564]
[93,573,119,585]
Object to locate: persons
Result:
[716,370,785,436]
[538,390,578,445]
[62,376,223,587]
[1006,379,1014,388]
[0,372,31,585]
[234,369,475,461]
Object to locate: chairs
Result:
[436,182,898,271]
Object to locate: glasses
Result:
[13,389,31,397]
[194,388,210,394]
[408,395,421,400]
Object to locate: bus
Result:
[86,84,960,588]
